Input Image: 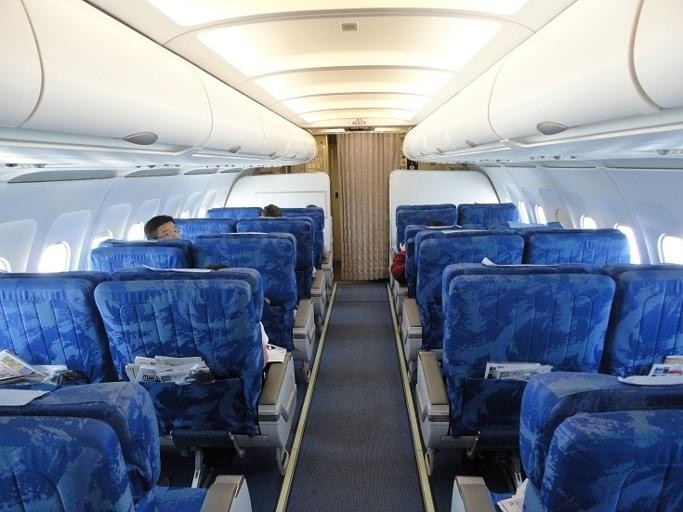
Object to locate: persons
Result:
[144,215,180,242]
[261,204,317,278]
[390,218,446,285]
[207,263,269,370]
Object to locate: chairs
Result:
[389,204,683,512]
[0,207,334,512]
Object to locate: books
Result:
[496,476,529,512]
[125,355,214,385]
[0,348,69,385]
[483,361,553,382]
[648,356,683,377]
[266,342,287,363]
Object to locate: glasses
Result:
[151,230,181,240]
